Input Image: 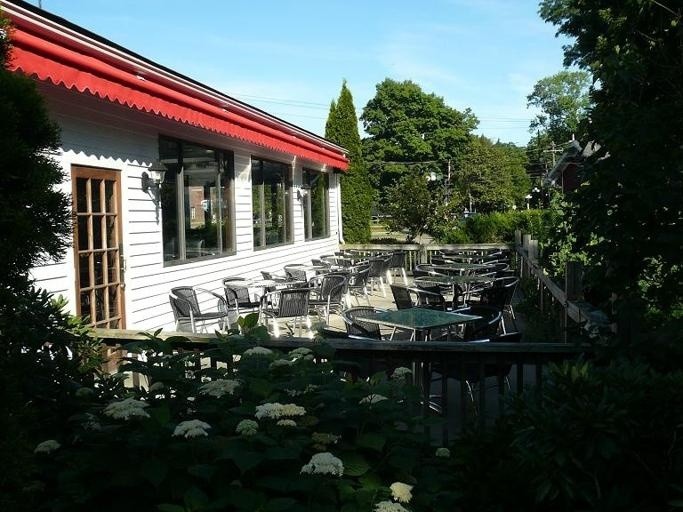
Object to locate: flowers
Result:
[170,311,423,424]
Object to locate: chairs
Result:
[170,285,228,333]
[390,248,524,423]
[283,247,392,311]
[262,288,313,340]
[221,277,262,331]
[262,270,287,295]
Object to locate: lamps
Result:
[141,159,168,206]
